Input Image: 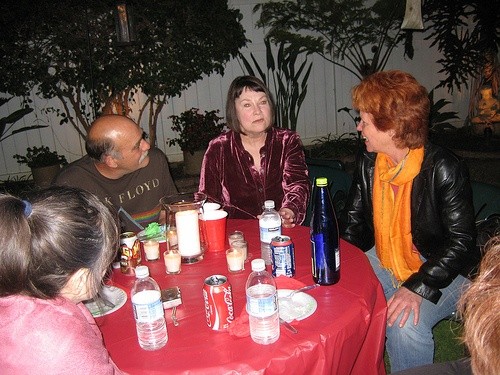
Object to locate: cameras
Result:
[161,286,182,309]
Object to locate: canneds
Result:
[119,231,142,274]
[270,235,296,278]
[201,274,234,332]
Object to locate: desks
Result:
[89,218,387,375]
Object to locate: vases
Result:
[183,149,204,175]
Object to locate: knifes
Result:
[279,317,298,334]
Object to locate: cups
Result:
[225,247,243,274]
[143,238,161,263]
[198,203,221,242]
[231,240,247,260]
[199,210,228,252]
[228,230,244,246]
[164,249,182,275]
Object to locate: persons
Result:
[195,76,308,228]
[0,186,122,375]
[53,114,179,235]
[339,70,482,372]
[386,234,500,375]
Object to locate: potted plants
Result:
[17,146,66,190]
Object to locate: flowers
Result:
[171,108,227,150]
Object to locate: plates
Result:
[137,225,176,243]
[246,288,317,323]
[83,285,127,318]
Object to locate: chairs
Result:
[378,180,500,372]
[305,158,352,227]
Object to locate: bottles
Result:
[245,259,280,345]
[310,177,341,286]
[258,200,281,266]
[159,191,208,266]
[130,265,168,352]
